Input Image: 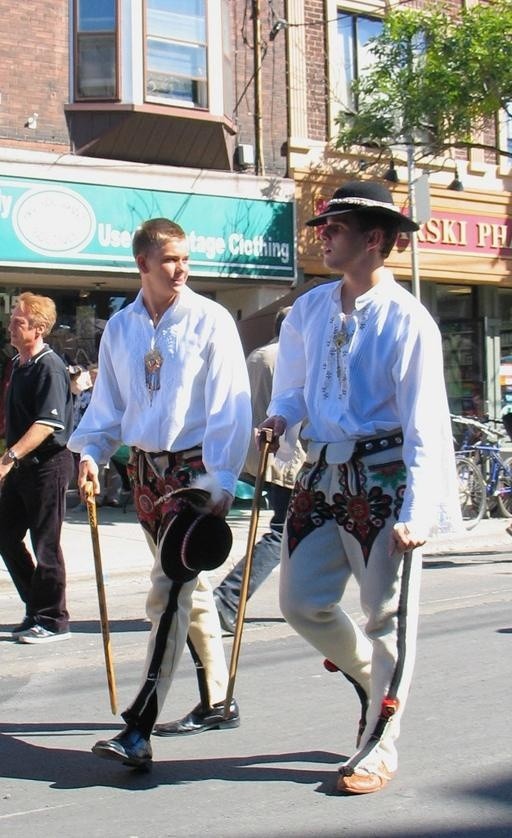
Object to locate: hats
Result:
[159,506,233,582]
[304,181,420,232]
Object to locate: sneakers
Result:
[11,616,71,643]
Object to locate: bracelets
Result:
[5,447,21,469]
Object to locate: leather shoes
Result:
[337,772,397,794]
[156,698,240,736]
[91,726,153,765]
[214,592,236,633]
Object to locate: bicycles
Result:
[450,413,512,531]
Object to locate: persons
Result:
[67,218,253,778]
[0,292,77,644]
[211,306,307,633]
[70,365,135,515]
[256,181,453,797]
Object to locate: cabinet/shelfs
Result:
[438,287,512,431]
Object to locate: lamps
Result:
[350,149,400,184]
[419,160,465,193]
